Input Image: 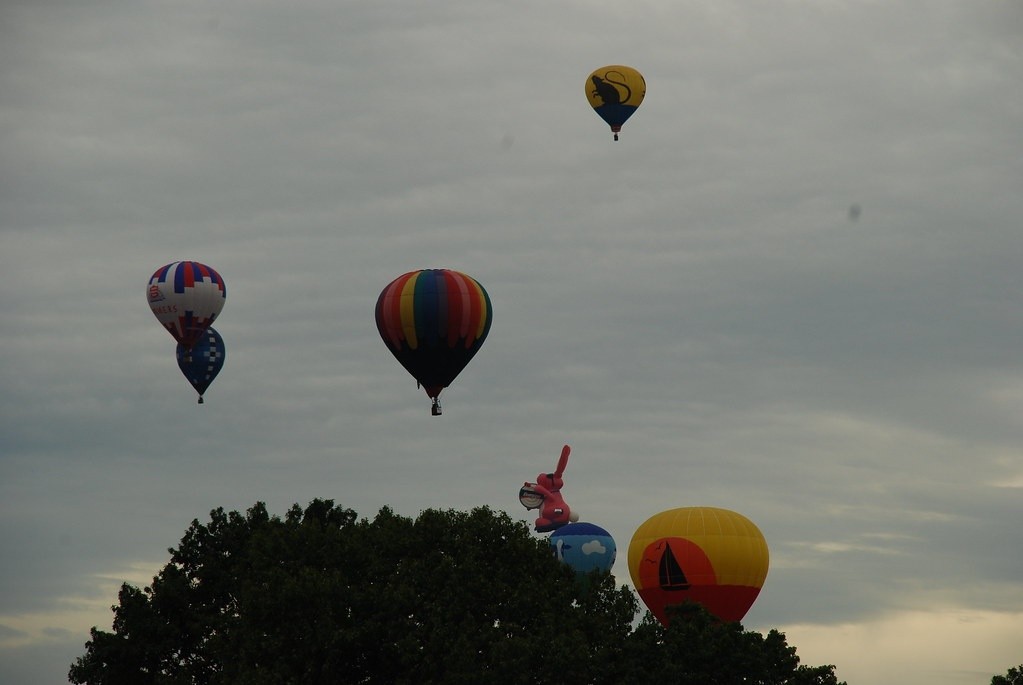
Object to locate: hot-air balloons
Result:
[146,260,226,361]
[547,522,616,612]
[374,269,493,416]
[176,326,226,405]
[586,65,646,141]
[628,507,770,630]
